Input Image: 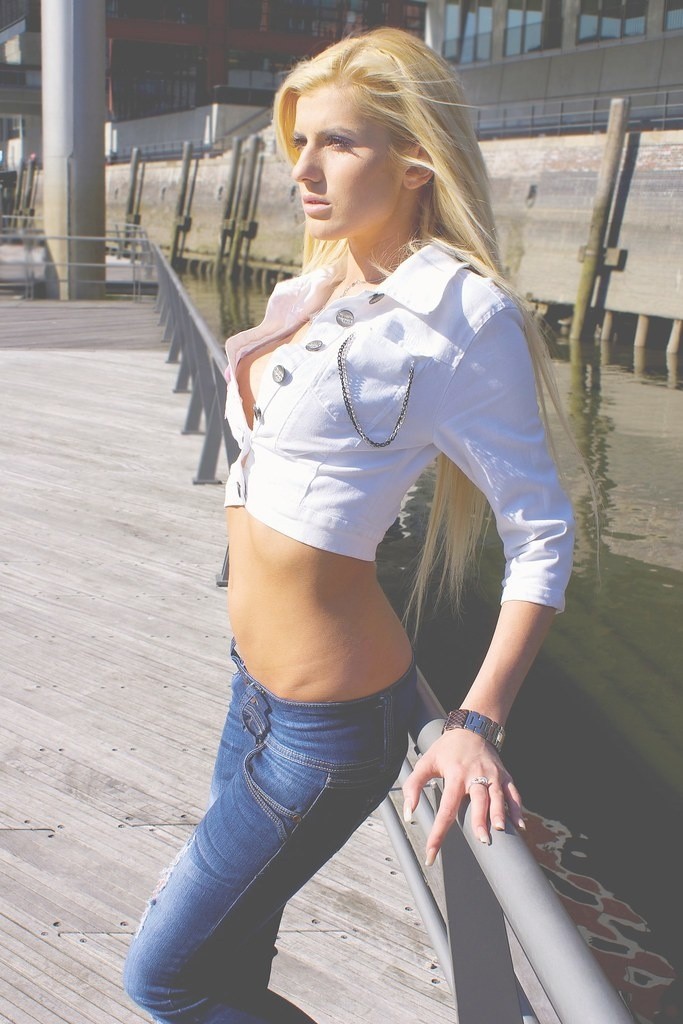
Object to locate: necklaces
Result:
[308,274,389,322]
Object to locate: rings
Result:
[468,775,490,791]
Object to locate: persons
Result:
[123,26,577,1024]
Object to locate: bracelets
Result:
[440,710,506,754]
